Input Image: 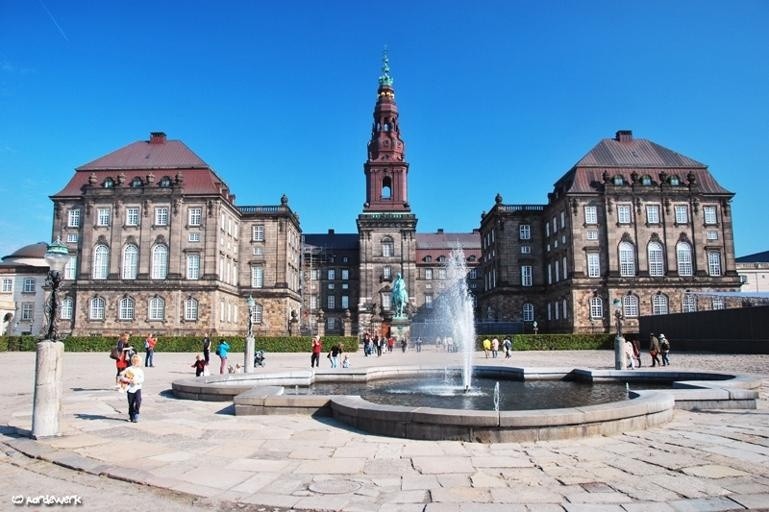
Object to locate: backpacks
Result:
[661,341,670,350]
[144,341,149,348]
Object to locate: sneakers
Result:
[129,413,140,422]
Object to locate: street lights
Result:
[244,293,256,373]
[614,297,627,370]
[30,235,70,439]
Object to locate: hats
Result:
[649,333,665,339]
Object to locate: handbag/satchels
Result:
[109,346,124,360]
[649,350,657,358]
